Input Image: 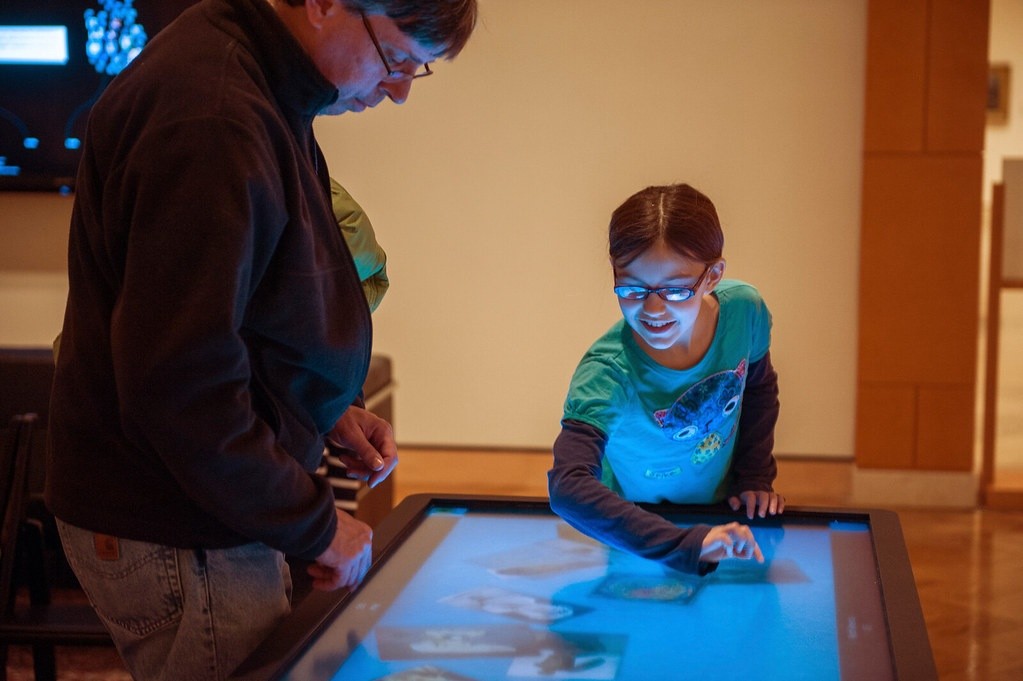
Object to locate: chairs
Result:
[0,350,115,681]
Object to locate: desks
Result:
[218,493,938,681]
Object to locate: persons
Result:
[546,180,774,578]
[44,1,480,681]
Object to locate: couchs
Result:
[0,355,393,642]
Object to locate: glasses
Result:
[353,6,434,81]
[613,263,711,302]
